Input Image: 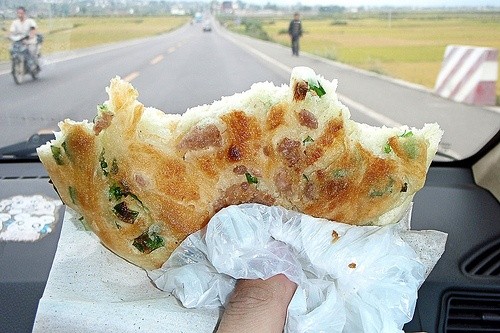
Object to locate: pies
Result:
[36,66,444,268]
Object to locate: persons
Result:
[214,244,298,333]
[289,13,302,55]
[8,6,40,73]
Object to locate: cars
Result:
[202,25,212,31]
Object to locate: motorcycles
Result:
[1,26,45,85]
